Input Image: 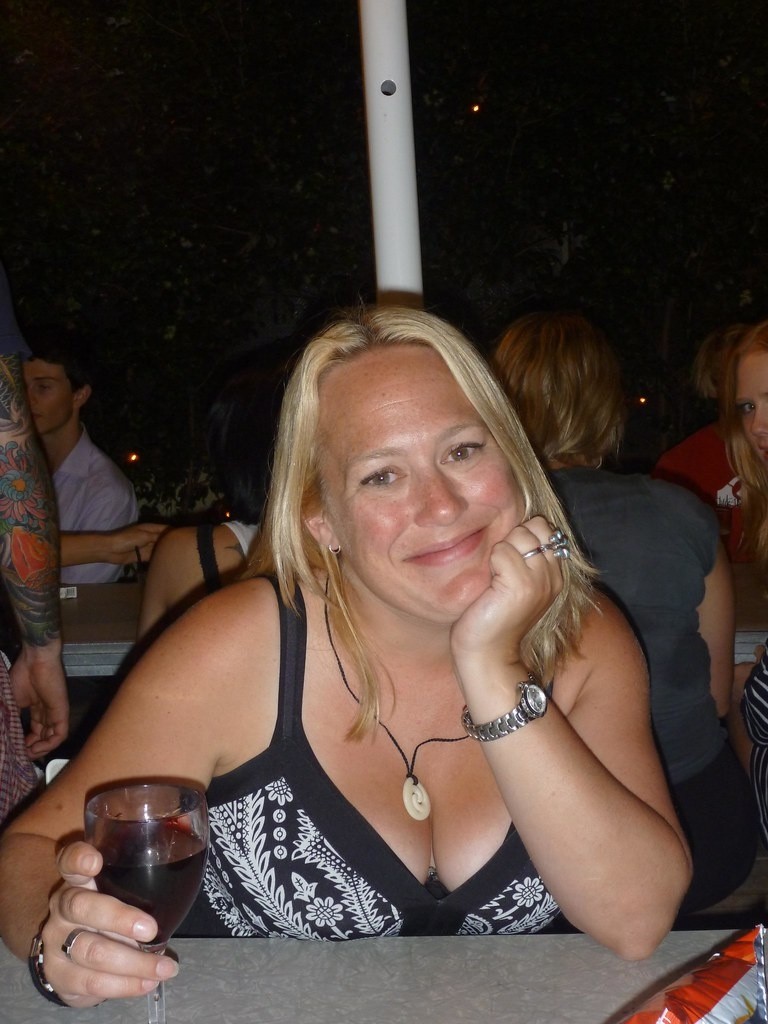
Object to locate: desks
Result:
[60,582,144,678]
[0,928,740,1024]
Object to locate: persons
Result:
[0,316,768,915]
[0,305,695,1010]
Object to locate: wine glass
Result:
[86,784,211,1024]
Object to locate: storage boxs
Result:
[60,585,81,599]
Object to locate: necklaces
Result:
[325,574,470,821]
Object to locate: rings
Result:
[520,547,544,560]
[61,927,84,961]
[541,529,570,558]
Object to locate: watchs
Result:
[461,674,550,742]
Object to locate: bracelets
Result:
[28,920,73,1007]
